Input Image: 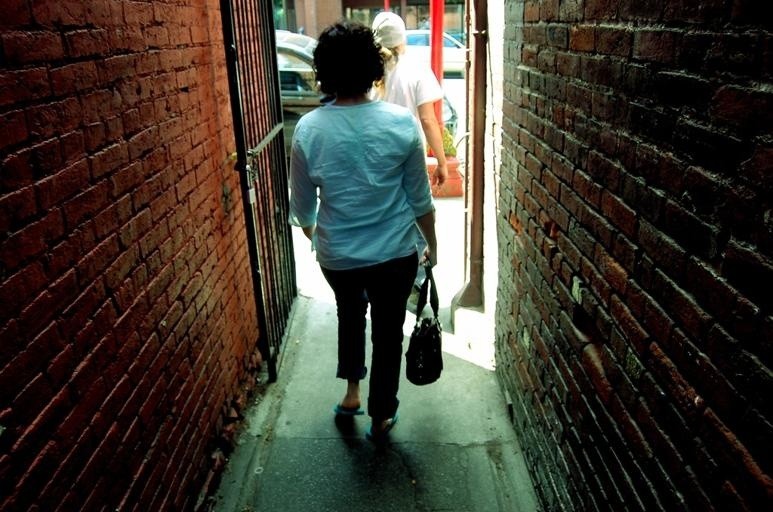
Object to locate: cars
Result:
[274,30,385,158]
[405,30,465,77]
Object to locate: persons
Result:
[286,21,438,444]
[364,12,448,315]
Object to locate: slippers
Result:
[332,403,365,416]
[365,414,398,442]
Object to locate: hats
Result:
[372,11,407,49]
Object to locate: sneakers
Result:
[405,288,426,316]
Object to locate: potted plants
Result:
[430,128,463,197]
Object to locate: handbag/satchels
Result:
[405,260,444,386]
